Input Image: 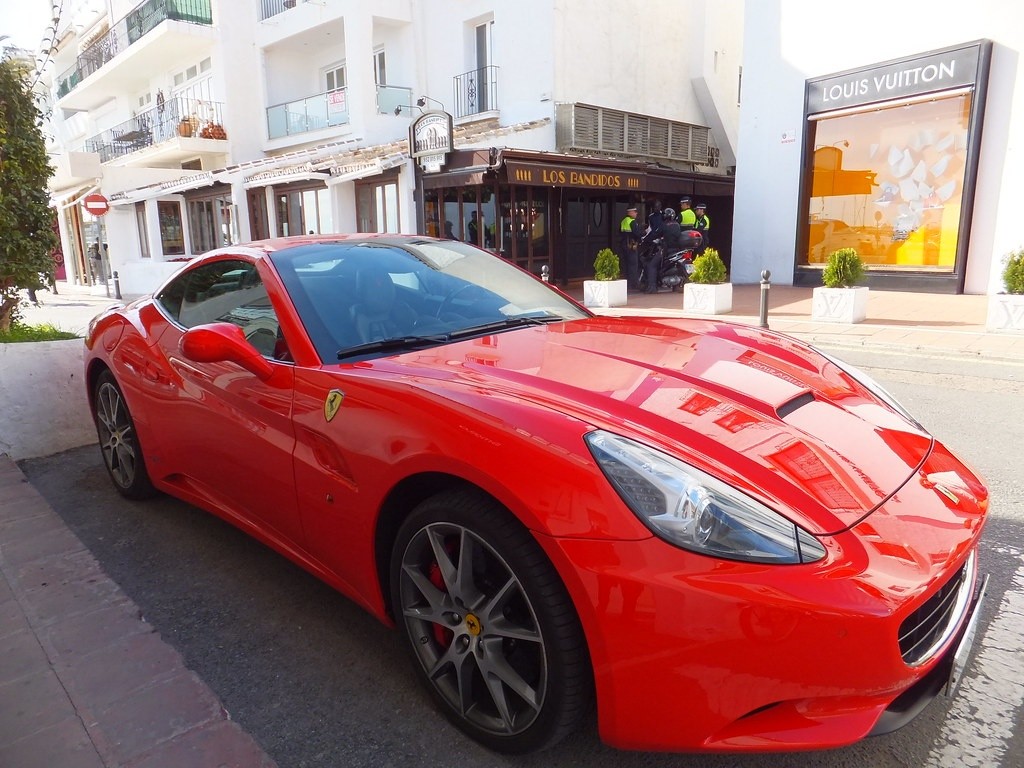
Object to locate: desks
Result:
[113,131,152,152]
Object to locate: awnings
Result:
[46,190,79,209]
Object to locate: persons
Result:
[445,211,492,246]
[91,237,108,284]
[620,196,709,294]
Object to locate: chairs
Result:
[353,265,417,343]
[112,129,152,155]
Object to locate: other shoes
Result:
[93,281,96,285]
[645,286,657,294]
[99,281,104,285]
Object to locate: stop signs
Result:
[84,194,109,216]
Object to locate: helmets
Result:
[660,207,675,223]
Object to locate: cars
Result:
[84,233,991,755]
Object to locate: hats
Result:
[626,206,637,211]
[693,203,707,210]
[676,196,693,205]
[471,210,478,216]
[445,221,453,226]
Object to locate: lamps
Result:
[394,105,424,116]
[417,95,444,111]
[833,139,849,148]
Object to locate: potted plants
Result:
[583,248,627,308]
[812,248,869,323]
[989,250,1024,329]
[683,247,733,315]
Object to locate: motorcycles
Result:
[636,230,703,294]
